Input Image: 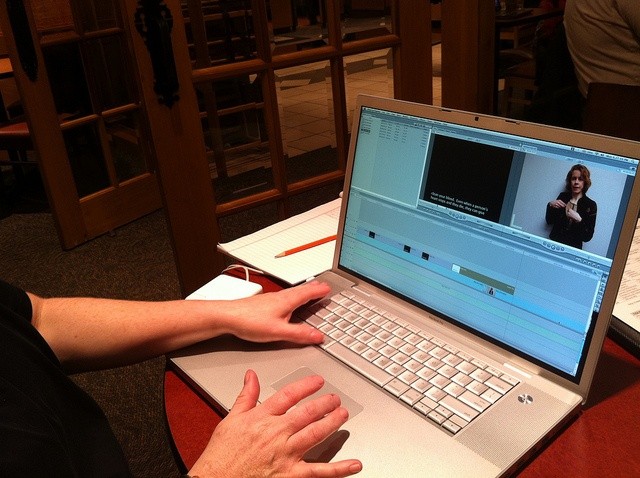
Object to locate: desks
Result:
[496,9,559,25]
[0,113,76,159]
[164,259,640,478]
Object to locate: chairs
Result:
[499,18,567,120]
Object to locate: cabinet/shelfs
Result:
[0,2,171,252]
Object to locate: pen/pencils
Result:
[274,234,337,258]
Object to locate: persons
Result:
[545,164,597,250]
[563,0,640,99]
[0,280,362,478]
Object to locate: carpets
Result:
[0,144,340,476]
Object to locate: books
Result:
[202,170,336,292]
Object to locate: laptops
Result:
[165,94,640,478]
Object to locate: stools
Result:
[501,31,518,48]
